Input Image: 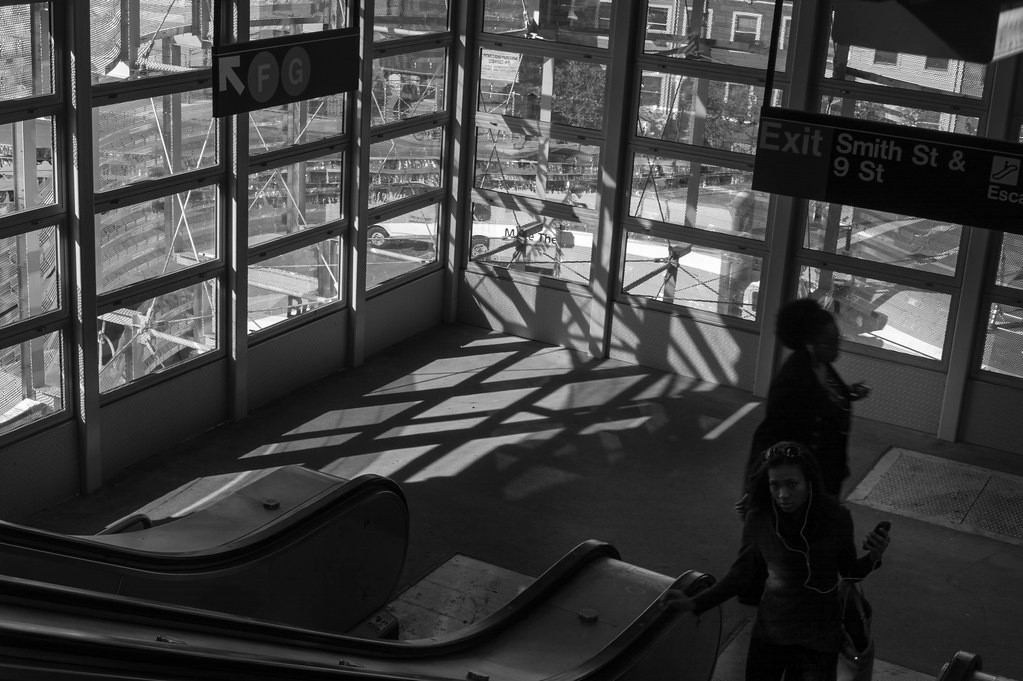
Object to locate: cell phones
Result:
[849,384,871,397]
[863,520,891,550]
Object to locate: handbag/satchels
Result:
[839,564,872,680]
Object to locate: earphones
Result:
[807,344,815,354]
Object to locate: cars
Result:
[363,195,498,259]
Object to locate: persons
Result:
[736,297,873,537]
[654,440,893,681]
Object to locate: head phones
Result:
[809,481,812,490]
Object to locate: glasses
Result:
[767,446,813,478]
